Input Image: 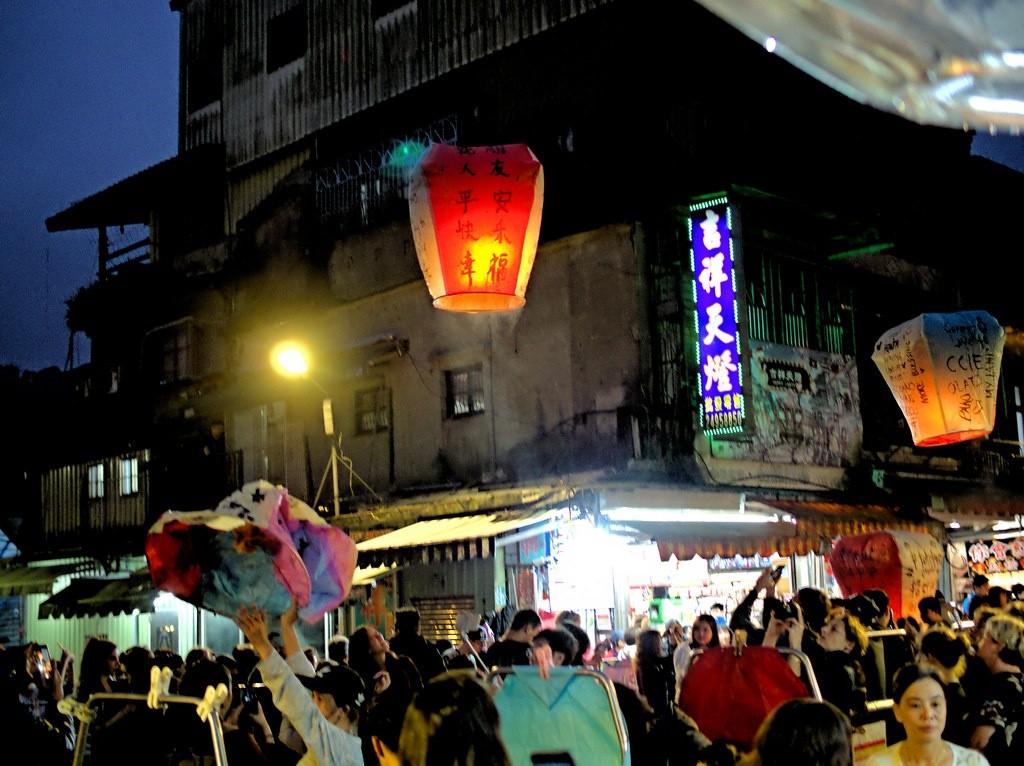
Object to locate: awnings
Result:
[0,564,77,597]
[750,498,928,540]
[896,478,1024,517]
[38,575,159,620]
[622,521,824,562]
[352,508,561,568]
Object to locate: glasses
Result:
[525,648,536,661]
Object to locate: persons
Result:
[0,566,1024,766]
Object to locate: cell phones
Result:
[771,566,784,580]
[38,646,52,672]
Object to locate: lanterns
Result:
[829,531,946,625]
[408,143,544,313]
[872,310,1005,448]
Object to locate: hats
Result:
[296,666,365,707]
[467,626,488,642]
[830,595,880,625]
